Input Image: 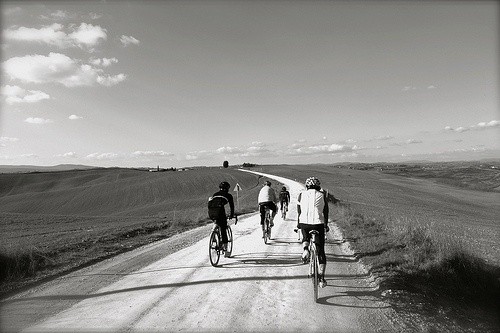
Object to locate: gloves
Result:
[229,216,233,220]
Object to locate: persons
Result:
[208,181,235,258]
[294,176,329,288]
[258,181,278,238]
[279,186,290,218]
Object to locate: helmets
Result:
[264,181,270,186]
[282,187,286,190]
[219,182,230,189]
[305,176,320,188]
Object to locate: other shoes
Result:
[286,207,288,211]
[301,250,309,263]
[224,251,230,256]
[281,216,283,218]
[320,281,327,288]
[270,218,273,225]
[261,225,265,231]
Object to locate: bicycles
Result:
[261,200,279,244]
[293,227,329,304]
[280,201,290,221]
[208,213,239,267]
